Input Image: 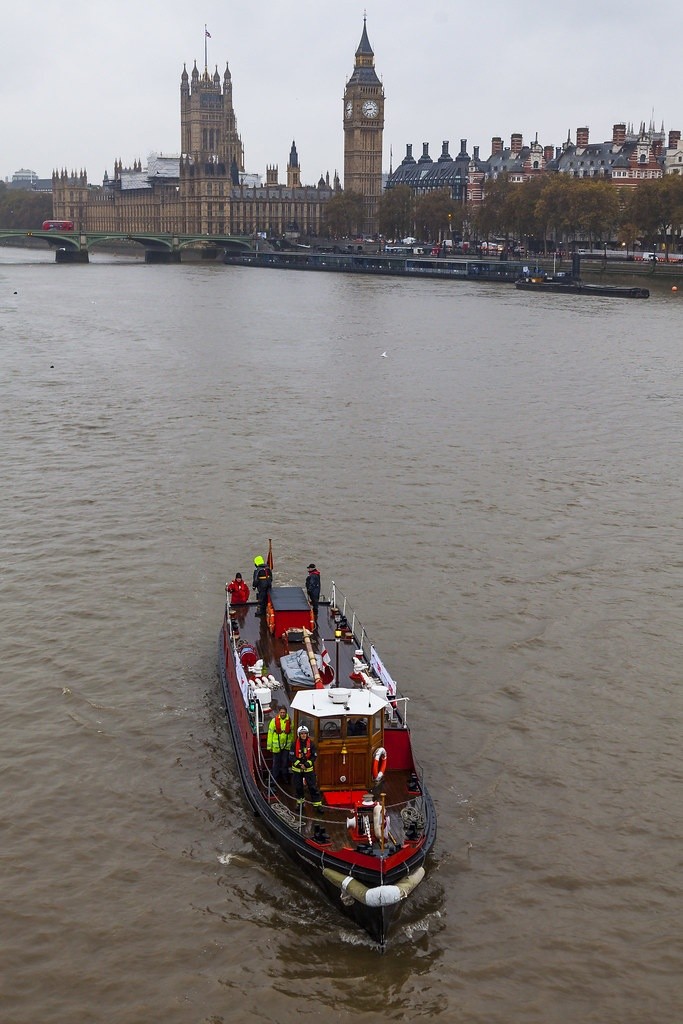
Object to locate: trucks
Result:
[569,249,586,256]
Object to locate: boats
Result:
[218,538,437,946]
[223,249,547,283]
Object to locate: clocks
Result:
[345,100,353,120]
[362,100,380,119]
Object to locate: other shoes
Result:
[297,805,304,811]
[271,778,279,784]
[283,778,289,784]
[316,806,324,813]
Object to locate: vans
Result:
[643,253,658,260]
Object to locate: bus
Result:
[42,220,74,231]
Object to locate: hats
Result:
[236,573,242,578]
[307,564,315,568]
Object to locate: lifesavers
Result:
[372,746,388,782]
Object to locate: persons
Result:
[289,726,327,814]
[252,556,273,616]
[228,572,250,604]
[265,707,295,783]
[305,563,321,615]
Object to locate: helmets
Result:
[297,726,309,739]
[254,556,264,567]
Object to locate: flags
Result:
[320,644,331,674]
[266,552,274,570]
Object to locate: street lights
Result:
[559,242,562,263]
[654,243,656,261]
[604,243,608,265]
[524,233,534,258]
[622,242,628,255]
